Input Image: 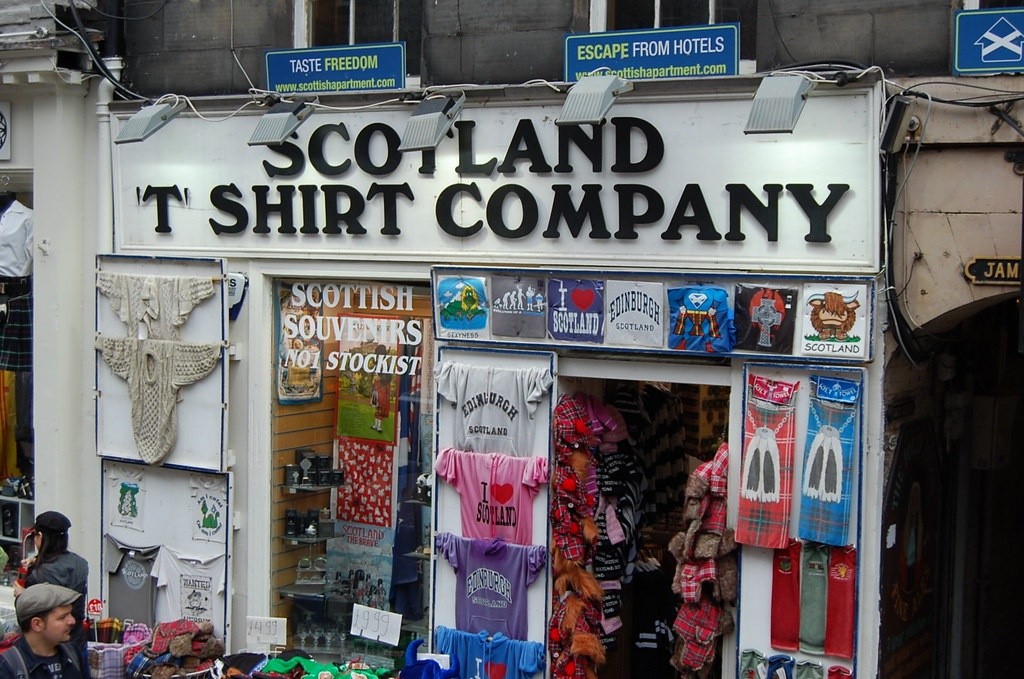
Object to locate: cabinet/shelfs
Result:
[277,484,350,601]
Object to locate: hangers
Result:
[0,175,16,213]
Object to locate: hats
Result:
[548,392,643,679]
[16,582,83,623]
[668,442,738,679]
[34,511,71,532]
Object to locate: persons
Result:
[369,346,393,431]
[0,192,35,370]
[12,511,90,679]
[0,583,85,679]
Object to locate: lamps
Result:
[398,89,466,152]
[113,93,188,145]
[245,95,322,146]
[743,74,821,133]
[557,75,635,125]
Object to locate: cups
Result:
[17,567,27,587]
